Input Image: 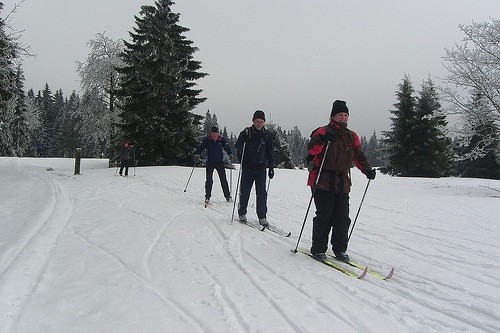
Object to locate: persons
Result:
[306,100,376,262]
[235,110,274,226]
[119,141,131,176]
[194,127,234,203]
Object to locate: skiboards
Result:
[297,247,395,281]
[234,218,292,237]
[122,175,134,178]
[204,199,255,208]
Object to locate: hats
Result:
[212,127,219,133]
[331,100,349,117]
[123,141,128,145]
[252,111,266,122]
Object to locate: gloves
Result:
[194,154,200,163]
[229,154,235,162]
[321,131,333,144]
[365,169,375,179]
[268,168,274,179]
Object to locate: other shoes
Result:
[313,252,326,259]
[335,251,348,259]
[240,215,247,222]
[205,198,209,203]
[260,218,268,225]
[226,197,232,202]
